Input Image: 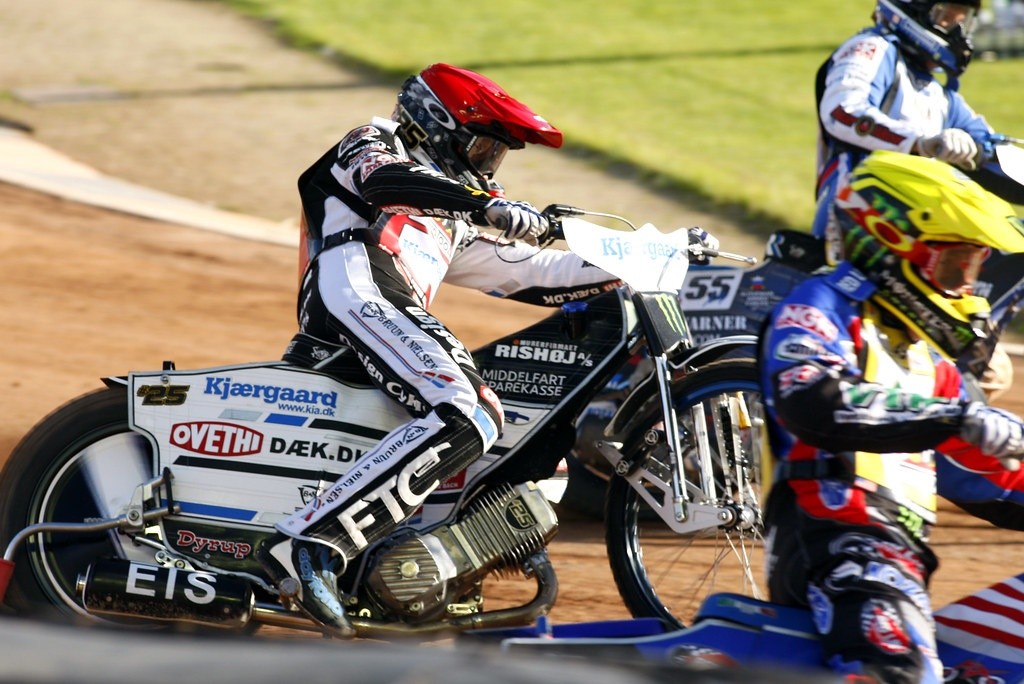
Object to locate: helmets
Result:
[827,147,1024,361]
[871,0,982,78]
[395,62,564,199]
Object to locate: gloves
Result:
[916,127,978,172]
[959,401,1024,471]
[481,198,551,246]
[688,226,721,264]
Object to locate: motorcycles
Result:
[1,201,798,648]
[442,421,1023,683]
[556,128,1024,530]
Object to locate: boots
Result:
[251,403,484,639]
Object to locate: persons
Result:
[254,63,718,637]
[815,0,1024,245]
[758,148,1024,684]
